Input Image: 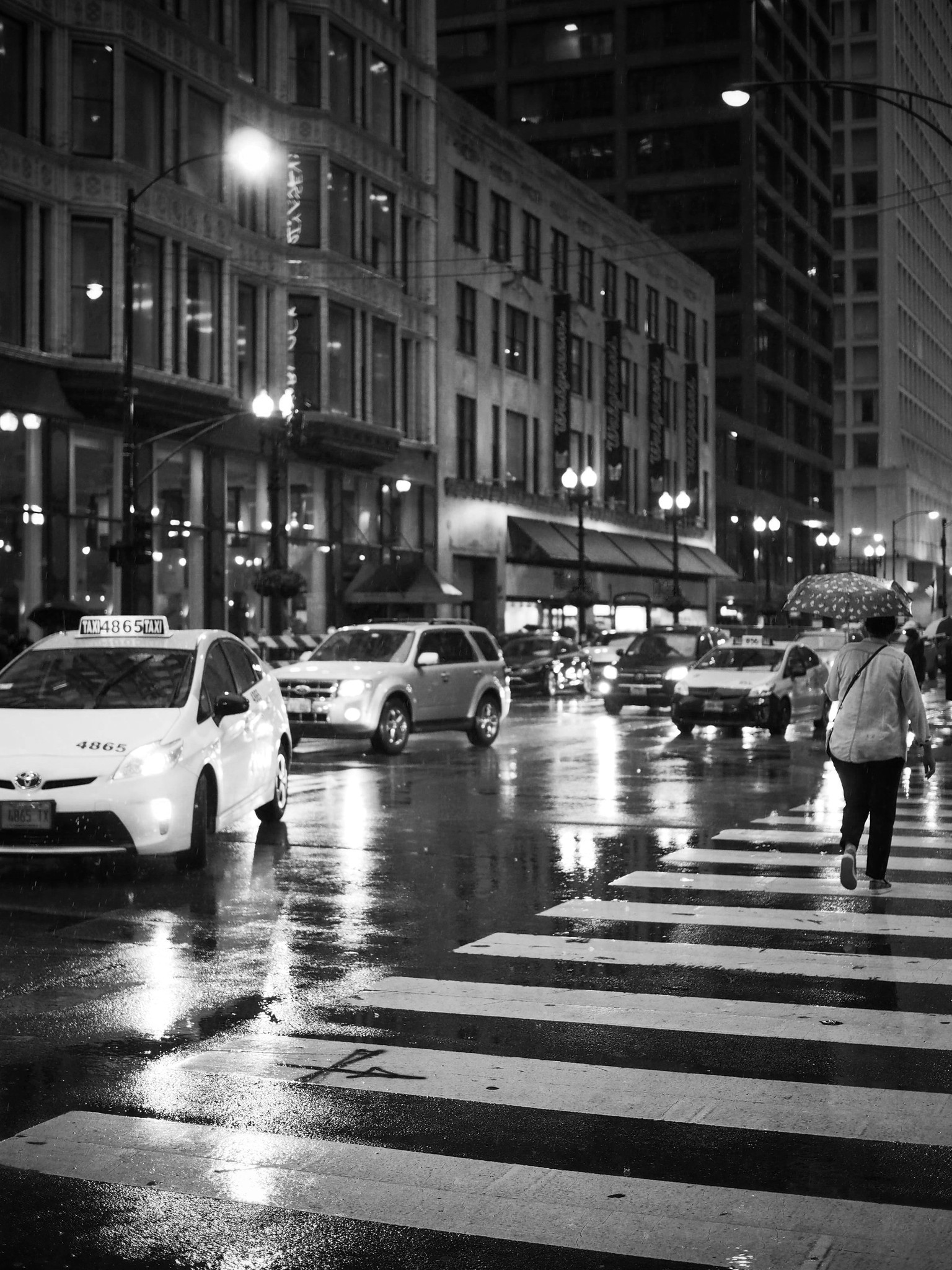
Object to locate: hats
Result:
[901,621,916,630]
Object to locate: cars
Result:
[0,613,294,872]
[793,615,951,679]
[495,624,646,699]
[669,635,832,737]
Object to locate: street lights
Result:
[561,465,599,647]
[848,526,863,571]
[892,510,939,581]
[864,543,886,576]
[658,489,690,624]
[814,532,841,629]
[873,532,887,578]
[117,123,278,614]
[753,515,781,626]
[249,387,301,660]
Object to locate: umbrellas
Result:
[781,572,914,647]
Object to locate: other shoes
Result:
[840,849,859,890]
[869,879,892,894]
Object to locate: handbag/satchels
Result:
[826,728,835,757]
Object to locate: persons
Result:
[824,615,936,894]
[900,621,927,687]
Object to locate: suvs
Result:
[598,624,730,716]
[271,617,511,755]
